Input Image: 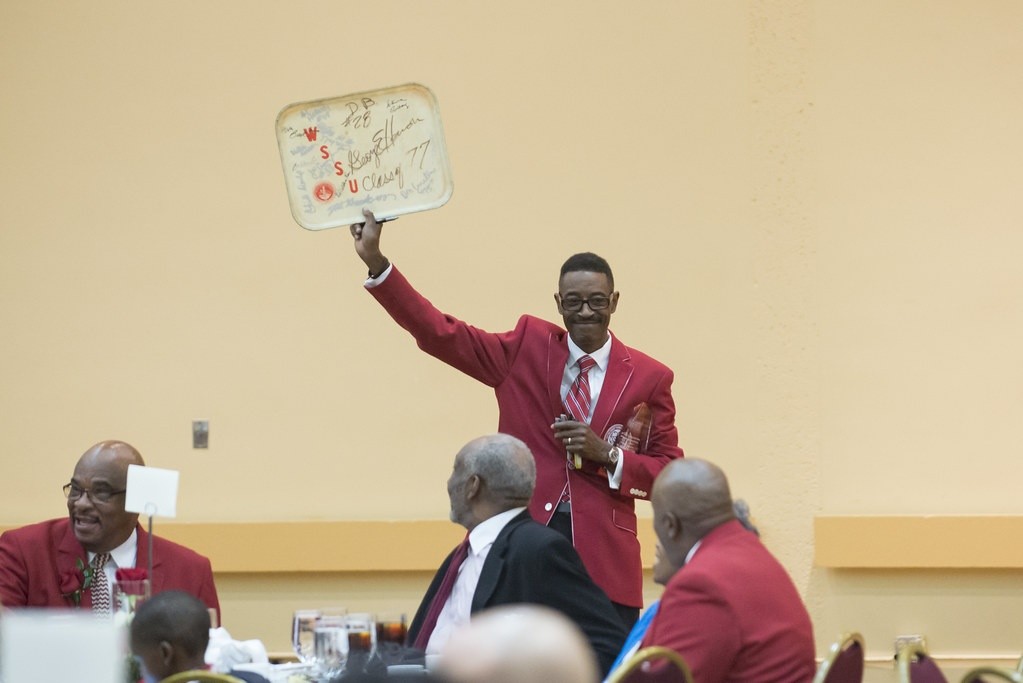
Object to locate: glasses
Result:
[558,292,612,312]
[61,482,126,502]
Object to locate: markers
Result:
[573,418,582,469]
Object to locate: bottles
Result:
[596,402,654,479]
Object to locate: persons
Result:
[635,456,816,682]
[428,604,600,683]
[400,433,629,680]
[0,441,222,629]
[605,498,761,682]
[352,209,684,633]
[130,595,273,683]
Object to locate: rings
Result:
[569,438,571,444]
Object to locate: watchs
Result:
[606,447,618,467]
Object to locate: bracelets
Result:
[368,256,387,279]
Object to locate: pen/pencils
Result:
[554,413,575,432]
[359,216,400,227]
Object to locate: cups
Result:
[375,611,408,646]
[315,607,349,683]
[891,632,931,683]
[346,612,371,652]
[111,579,153,621]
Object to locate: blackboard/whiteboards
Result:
[275,82,454,232]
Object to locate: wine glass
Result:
[290,609,323,674]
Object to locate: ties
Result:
[90,553,112,623]
[559,354,598,503]
[411,530,473,653]
